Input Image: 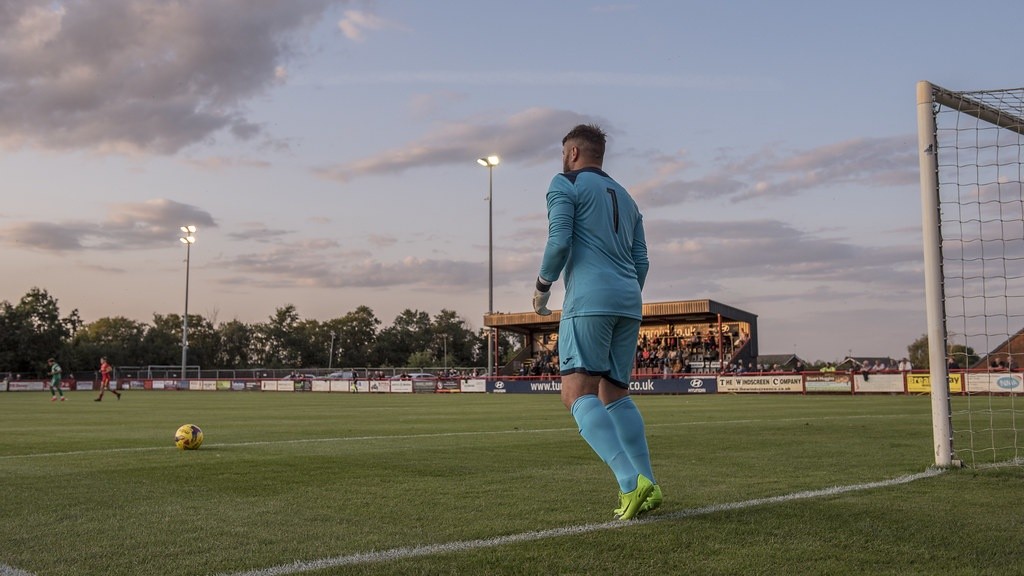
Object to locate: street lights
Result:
[478,156,500,378]
[329,330,336,368]
[179,225,197,379]
[437,333,447,374]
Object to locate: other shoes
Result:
[117,394,121,400]
[60,396,64,401]
[94,399,101,401]
[50,395,57,400]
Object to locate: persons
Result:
[1004,357,1019,397]
[69,373,76,390]
[948,358,960,372]
[792,361,804,375]
[17,374,22,380]
[820,362,836,377]
[256,371,261,378]
[290,326,748,380]
[989,357,1006,372]
[770,364,784,375]
[871,359,885,374]
[860,360,872,372]
[748,363,756,376]
[898,357,912,374]
[533,124,662,520]
[94,356,121,401]
[885,359,898,374]
[8,371,13,382]
[759,365,767,376]
[47,358,65,401]
[848,360,860,371]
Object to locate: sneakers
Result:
[636,483,662,518]
[613,474,654,521]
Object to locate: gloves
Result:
[532,276,553,316]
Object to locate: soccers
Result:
[175,424,204,450]
